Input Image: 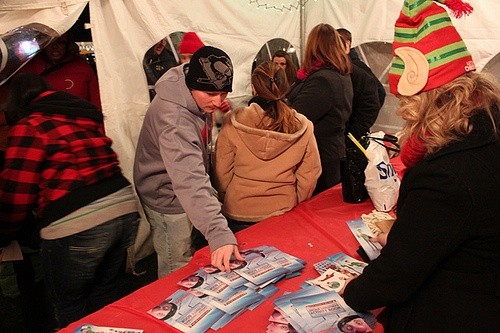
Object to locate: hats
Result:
[186,46,233,92]
[179,32,204,54]
[388,0,476,98]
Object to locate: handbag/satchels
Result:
[340,131,401,213]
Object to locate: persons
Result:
[143,38,179,103]
[27,32,105,135]
[213,59,323,232]
[177,31,233,145]
[337,0,500,333]
[133,46,246,280]
[271,49,298,86]
[283,23,386,191]
[0,69,137,328]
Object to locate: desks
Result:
[56,156,405,333]
